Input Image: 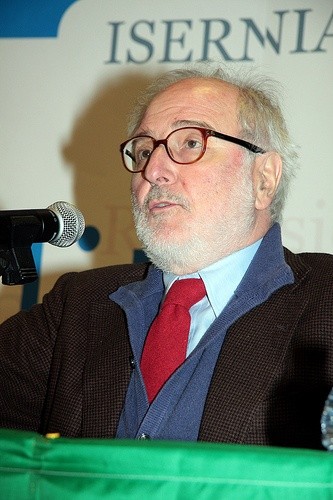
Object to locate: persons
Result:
[1,62,333,451]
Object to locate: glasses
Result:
[119,127,266,173]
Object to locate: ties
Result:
[137,278,207,407]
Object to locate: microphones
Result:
[1,200,86,246]
